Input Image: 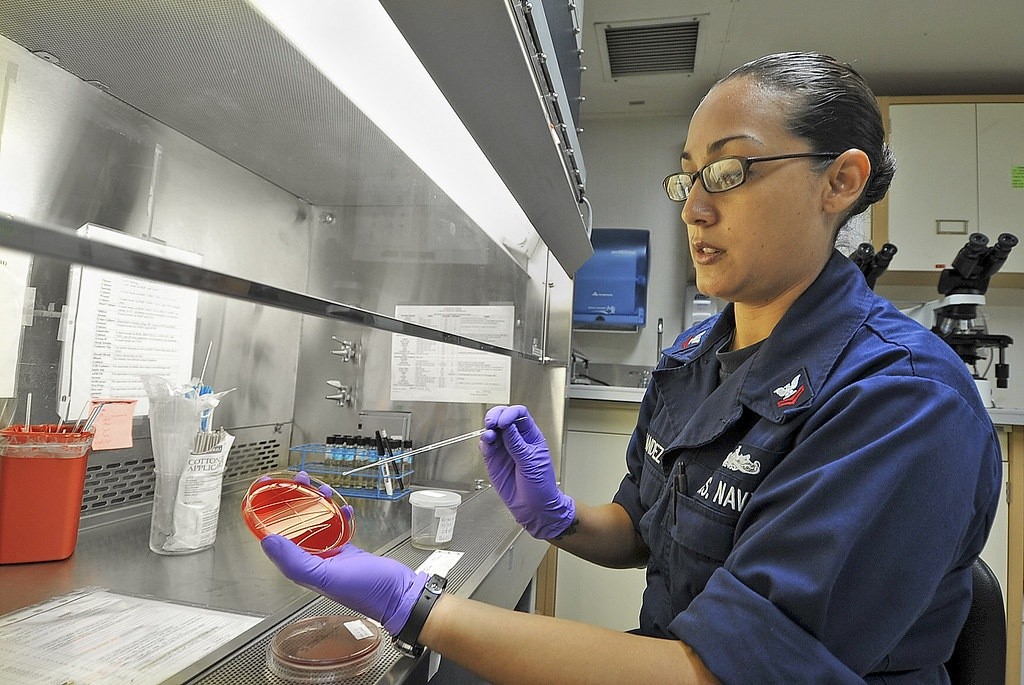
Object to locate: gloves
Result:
[257,471,429,637]
[478,405,576,541]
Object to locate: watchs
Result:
[389,575,448,659]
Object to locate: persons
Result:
[260,43,1002,685]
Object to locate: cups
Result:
[409,490,461,550]
[149,467,227,555]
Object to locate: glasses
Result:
[661,152,841,201]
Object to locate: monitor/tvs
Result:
[572,227,650,324]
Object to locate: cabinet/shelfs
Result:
[871,94,1023,289]
[534,384,1024,683]
